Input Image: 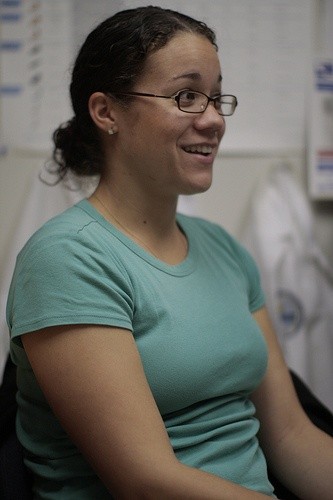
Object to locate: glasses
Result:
[103,88,238,117]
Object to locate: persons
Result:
[6,4,333,500]
